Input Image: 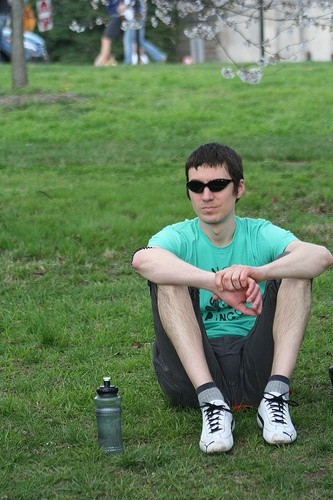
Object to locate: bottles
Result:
[94,377,124,455]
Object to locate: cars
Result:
[0,13,48,63]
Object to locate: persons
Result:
[131,143,333,453]
[90,0,167,67]
